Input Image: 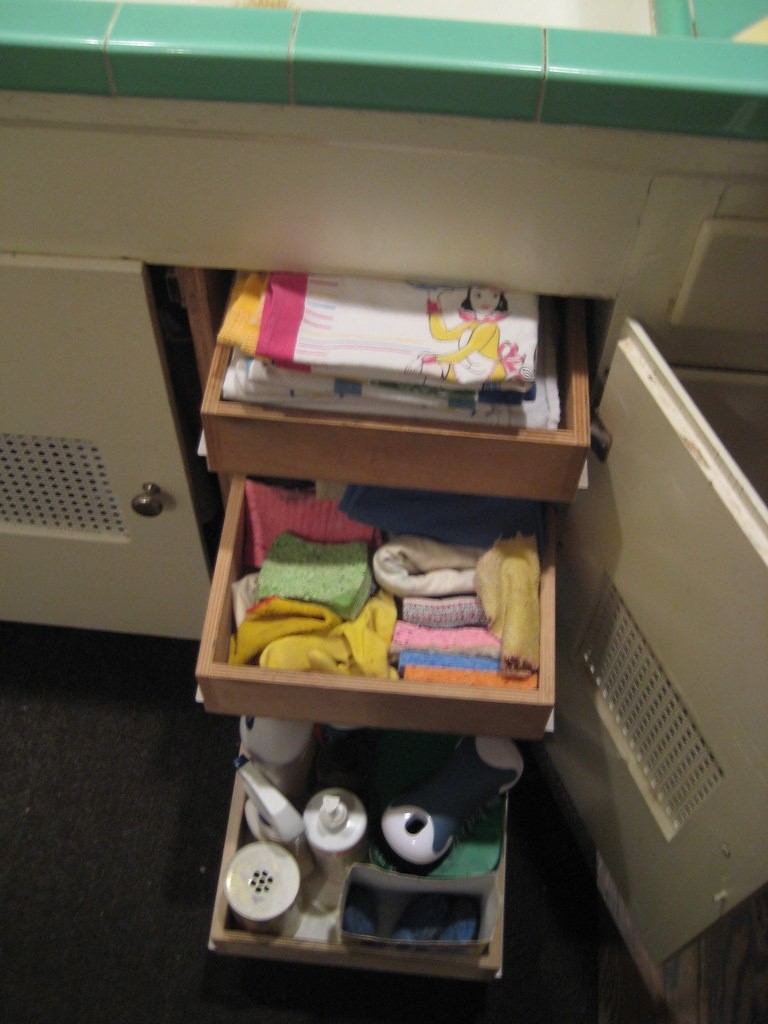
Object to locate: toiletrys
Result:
[231,753,372,883]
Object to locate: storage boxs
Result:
[336,862,500,955]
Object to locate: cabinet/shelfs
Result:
[0,92,768,1002]
